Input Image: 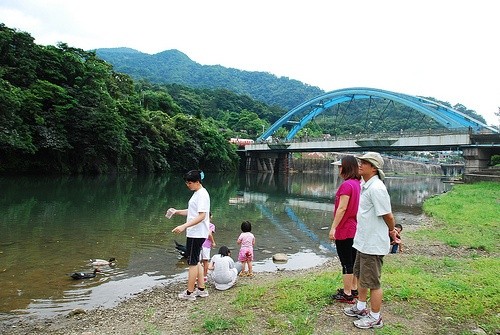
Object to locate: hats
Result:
[330,159,342,166]
[357,151,384,169]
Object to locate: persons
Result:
[389,223,403,254]
[208,246,242,290]
[344,151,402,329]
[237,220,255,277]
[166,170,209,300]
[326,155,361,303]
[199,212,216,283]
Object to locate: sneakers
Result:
[193,288,208,297]
[343,304,368,318]
[352,312,383,329]
[331,289,358,303]
[178,290,196,301]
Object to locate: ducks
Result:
[88,257,119,266]
[65,268,102,278]
[174,239,186,257]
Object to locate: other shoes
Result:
[239,272,253,277]
